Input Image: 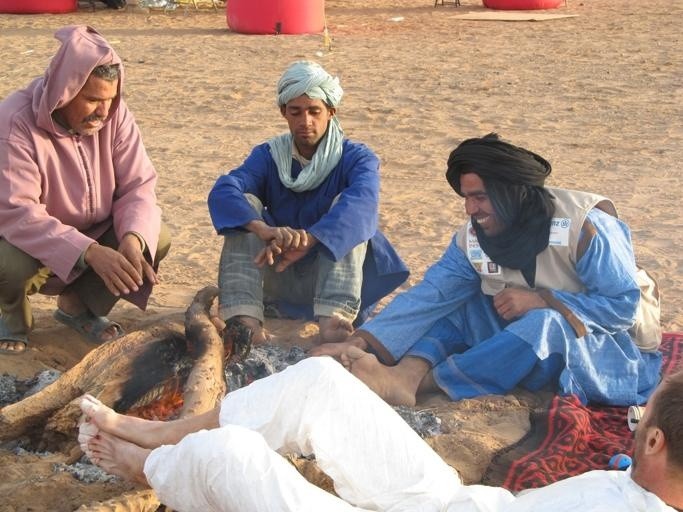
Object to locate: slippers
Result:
[0,333,30,354]
[53,308,125,344]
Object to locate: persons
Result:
[77,356,683,512]
[1,24,173,355]
[208,59,411,346]
[309,132,663,406]
